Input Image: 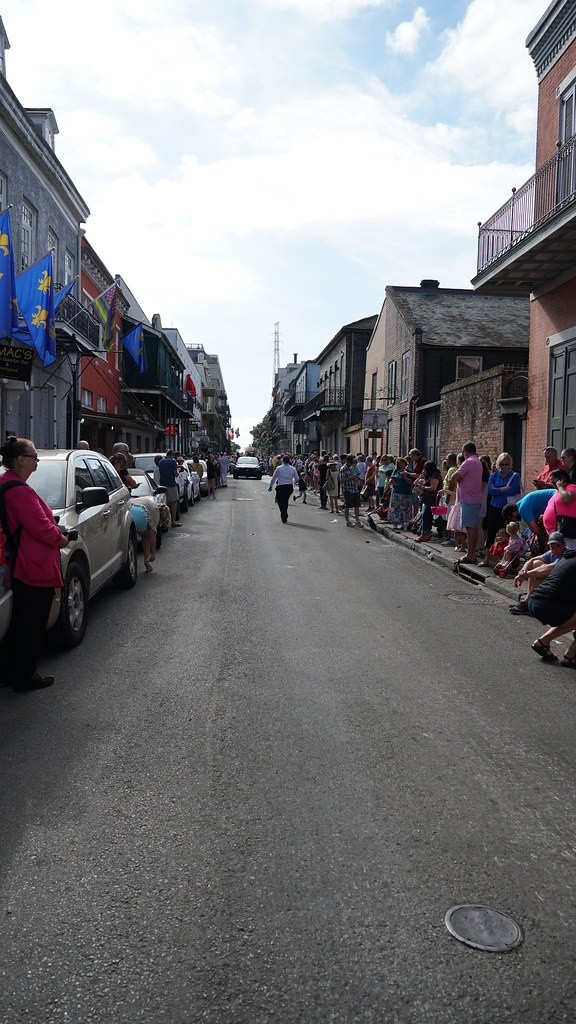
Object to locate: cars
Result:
[185,459,208,497]
[127,466,167,552]
[232,456,263,480]
[182,460,201,507]
[0,449,138,651]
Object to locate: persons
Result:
[0,437,70,691]
[132,499,171,572]
[528,550,576,664]
[292,448,467,552]
[452,442,483,564]
[154,449,240,527]
[247,451,283,476]
[478,446,576,615]
[77,440,104,455]
[109,443,138,493]
[269,456,298,524]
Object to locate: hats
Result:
[547,532,565,545]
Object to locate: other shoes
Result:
[346,522,354,527]
[454,546,465,552]
[478,561,489,567]
[508,601,529,614]
[460,556,478,564]
[415,534,430,541]
[355,522,364,528]
[366,507,375,512]
[11,672,54,691]
[146,564,152,573]
[173,522,182,527]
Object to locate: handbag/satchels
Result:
[506,473,525,504]
[323,469,335,491]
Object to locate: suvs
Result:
[131,452,189,522]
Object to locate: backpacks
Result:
[0,480,29,579]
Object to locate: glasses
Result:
[16,454,38,461]
[499,463,508,466]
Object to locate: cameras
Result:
[53,515,78,542]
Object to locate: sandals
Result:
[561,655,576,667]
[531,639,558,661]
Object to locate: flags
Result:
[16,251,57,367]
[92,285,117,355]
[12,279,76,349]
[0,211,19,338]
[122,324,147,372]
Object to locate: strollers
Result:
[410,492,444,536]
[493,521,542,579]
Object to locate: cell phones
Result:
[559,476,566,483]
[533,480,539,483]
